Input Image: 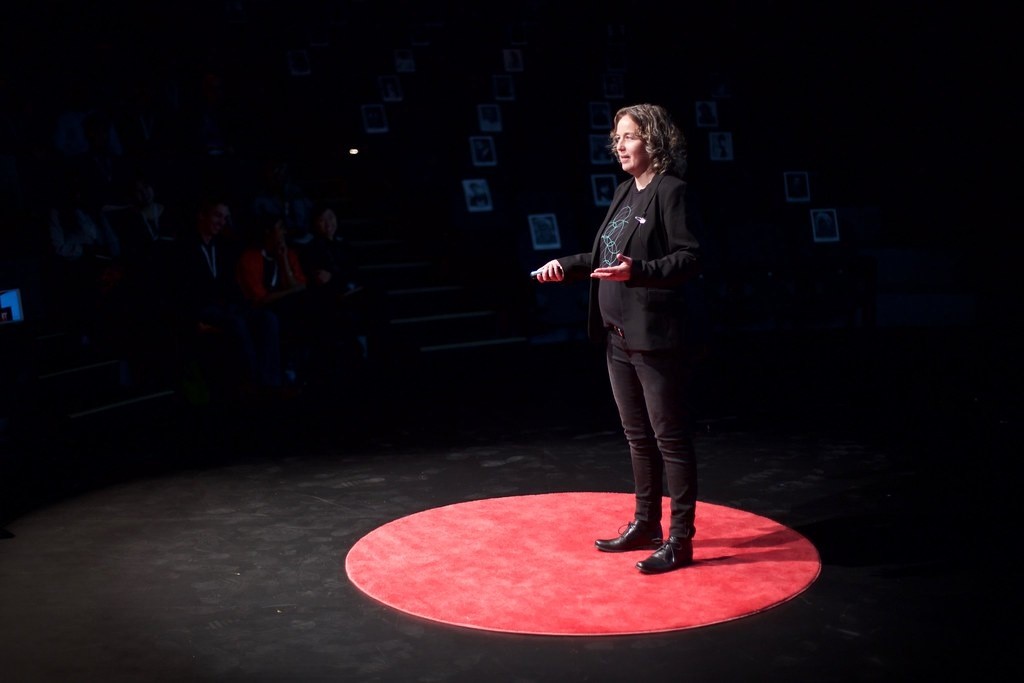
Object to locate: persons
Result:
[301,197,371,380]
[234,212,309,401]
[162,195,264,420]
[528,103,705,575]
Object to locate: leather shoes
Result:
[635,536,693,571]
[595,520,664,552]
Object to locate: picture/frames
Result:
[394,48,416,72]
[288,49,312,76]
[709,131,735,161]
[478,104,504,133]
[783,171,811,203]
[492,75,516,101]
[588,134,614,165]
[591,174,617,207]
[503,49,524,72]
[602,71,625,98]
[528,212,562,251]
[361,103,389,134]
[461,178,494,213]
[810,207,840,244]
[379,75,404,102]
[469,135,498,168]
[589,102,612,130]
[696,101,719,128]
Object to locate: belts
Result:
[611,325,624,336]
